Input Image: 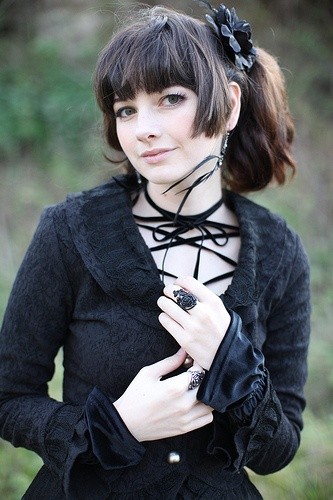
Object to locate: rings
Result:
[186,369,205,389]
[170,288,197,311]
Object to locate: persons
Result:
[0,5,312,500]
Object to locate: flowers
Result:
[197,0,258,72]
